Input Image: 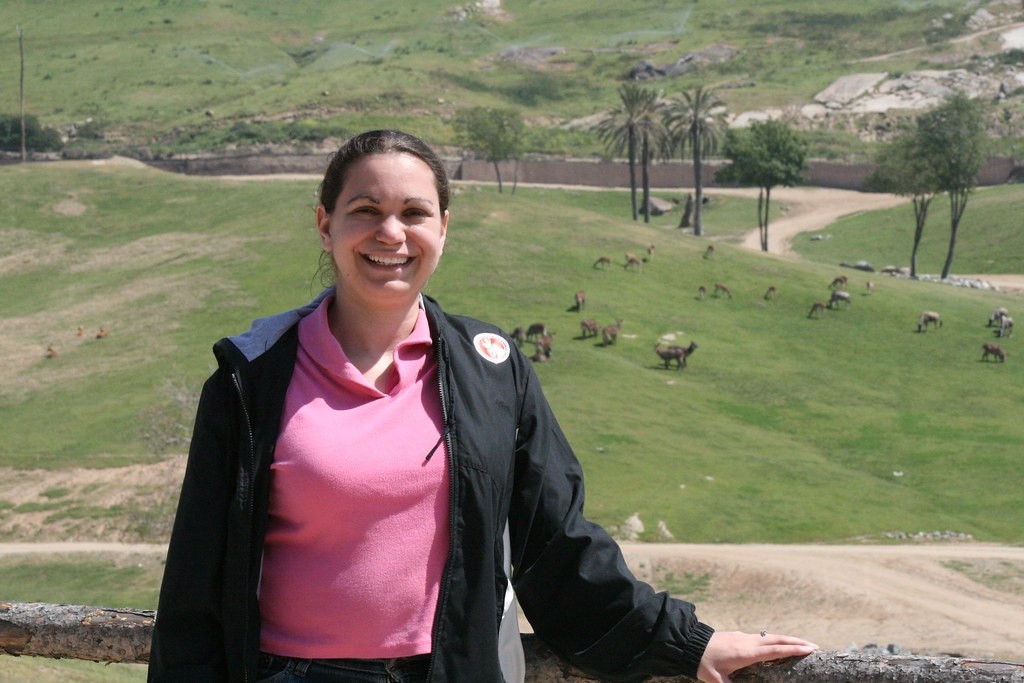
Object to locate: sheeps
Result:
[807,275,874,318]
[981,342,1006,363]
[603,317,624,346]
[580,320,600,337]
[703,245,714,258]
[575,290,585,313]
[696,283,733,299]
[653,340,698,371]
[509,322,556,364]
[917,310,940,332]
[46,325,107,359]
[765,285,777,300]
[592,242,656,273]
[988,306,1013,338]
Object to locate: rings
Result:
[760,630,767,637]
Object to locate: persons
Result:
[147,131,819,683]
